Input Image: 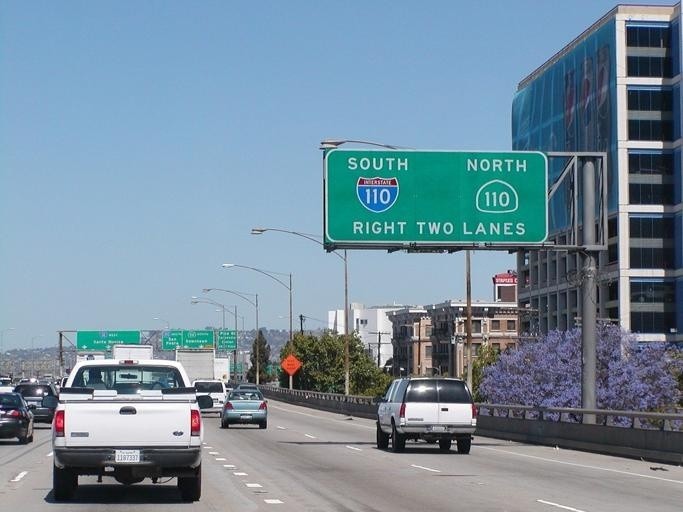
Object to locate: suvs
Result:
[371,376,479,455]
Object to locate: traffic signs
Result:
[321,146,550,252]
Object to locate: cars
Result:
[219,387,269,429]
[191,379,227,418]
[0,373,59,445]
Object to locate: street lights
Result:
[251,224,349,396]
[200,287,260,387]
[217,263,293,358]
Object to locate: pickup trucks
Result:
[40,359,214,500]
